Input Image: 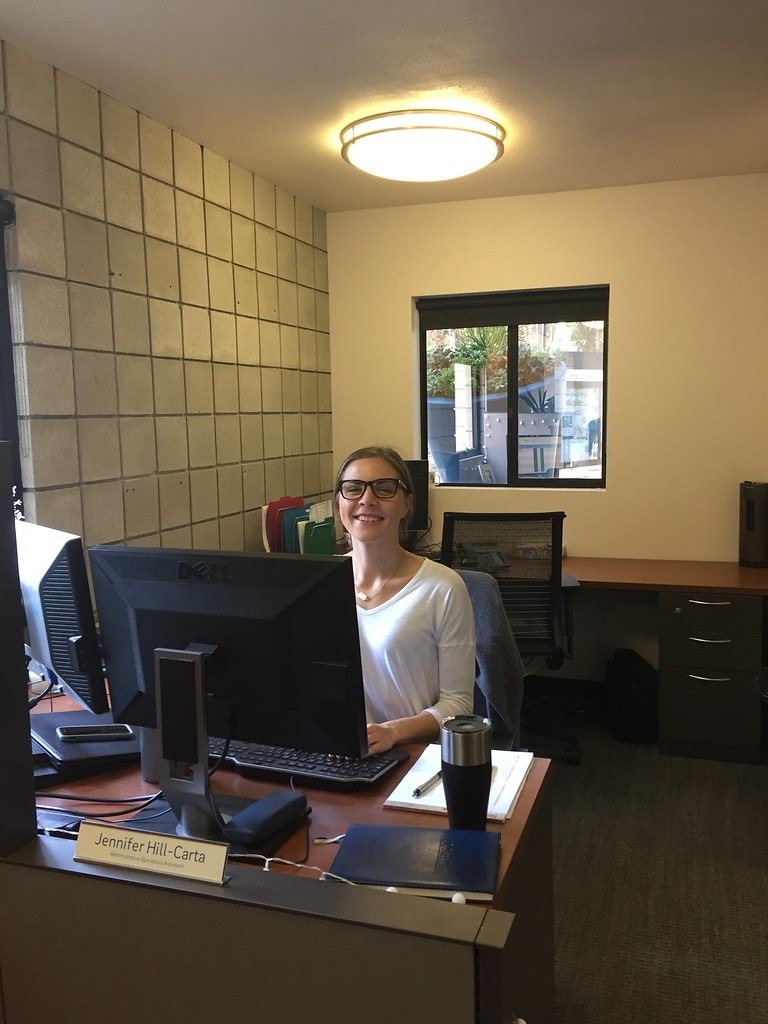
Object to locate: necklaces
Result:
[355,549,404,601]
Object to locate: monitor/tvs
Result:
[402,460,429,532]
[14,519,369,864]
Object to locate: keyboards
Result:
[208,733,410,792]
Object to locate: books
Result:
[381,744,534,822]
[258,496,332,554]
[328,822,501,903]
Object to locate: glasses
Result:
[335,478,410,501]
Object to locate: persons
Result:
[336,447,476,758]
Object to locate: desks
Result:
[0,743,553,1024]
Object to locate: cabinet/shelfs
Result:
[529,557,768,764]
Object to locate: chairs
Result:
[440,510,595,761]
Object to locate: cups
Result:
[439,715,492,832]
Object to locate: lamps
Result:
[341,108,507,182]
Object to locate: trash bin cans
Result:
[755,668,768,759]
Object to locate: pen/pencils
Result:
[412,771,442,797]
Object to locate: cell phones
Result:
[56,724,134,742]
